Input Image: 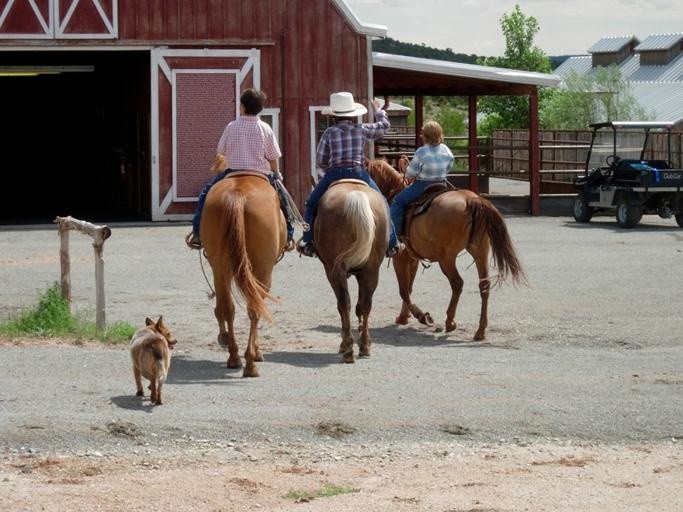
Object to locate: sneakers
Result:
[391,242,406,257]
[189,237,200,245]
[297,239,313,257]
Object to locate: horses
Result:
[306,173,391,363]
[198,153,288,379]
[362,155,532,342]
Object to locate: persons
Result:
[391,120,454,234]
[189,88,295,247]
[296,91,405,258]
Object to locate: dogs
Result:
[130,315,180,407]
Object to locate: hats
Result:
[319,90,367,121]
